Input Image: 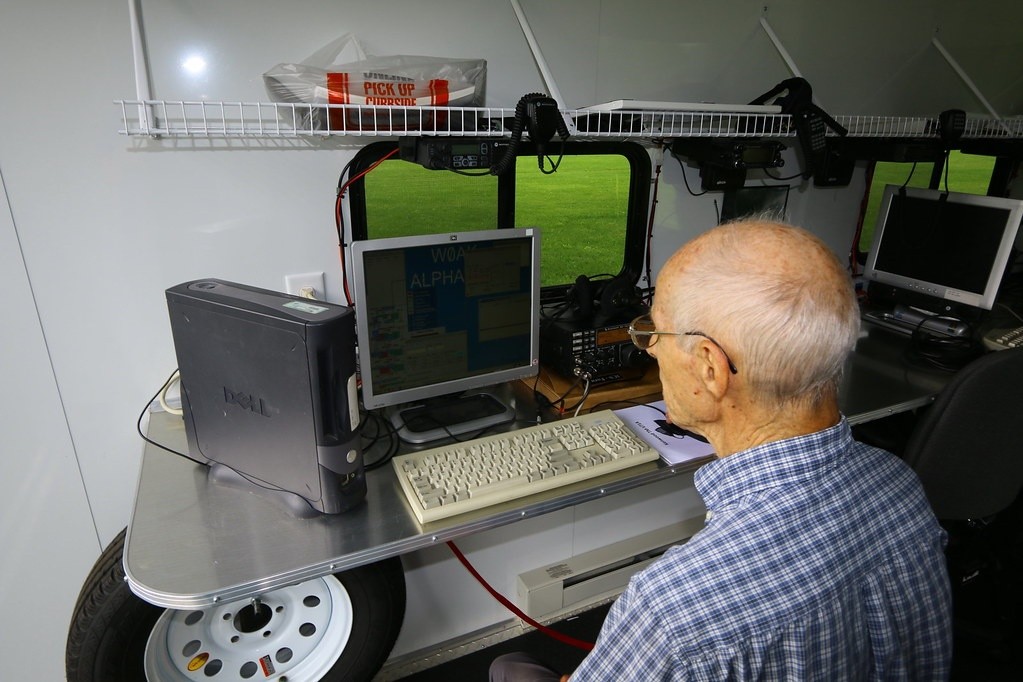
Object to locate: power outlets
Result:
[286,271,327,305]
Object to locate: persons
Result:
[488,210,955,682]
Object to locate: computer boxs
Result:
[166,278,368,514]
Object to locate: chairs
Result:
[855,346,1023,587]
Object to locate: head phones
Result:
[566,274,657,323]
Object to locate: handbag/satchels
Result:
[264,33,488,131]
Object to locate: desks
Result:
[122,358,932,682]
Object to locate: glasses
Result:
[627,311,738,375]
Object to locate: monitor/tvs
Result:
[351,226,540,443]
[862,184,1023,338]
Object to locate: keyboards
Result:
[981,321,1023,352]
[392,409,660,524]
